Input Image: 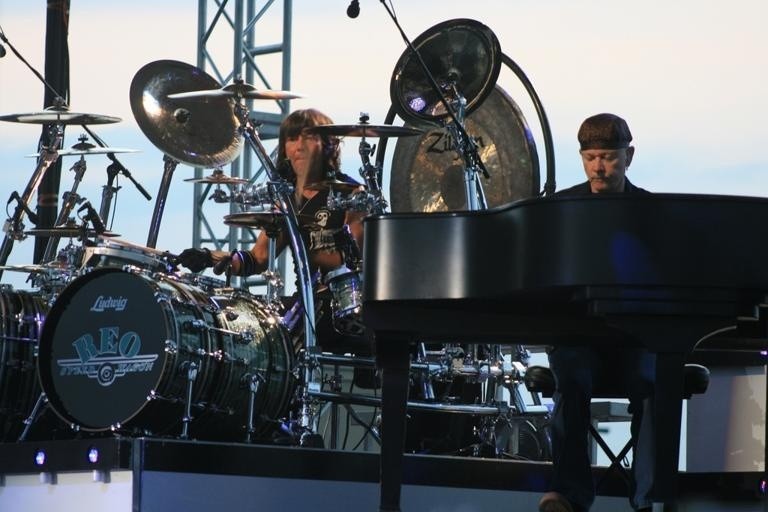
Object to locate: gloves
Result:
[173,247,213,273]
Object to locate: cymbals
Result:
[0,262,76,272]
[0,113,121,125]
[222,213,319,225]
[185,178,253,184]
[390,19,501,125]
[304,124,425,135]
[20,229,121,237]
[302,179,363,193]
[24,148,138,154]
[129,59,242,168]
[166,84,302,100]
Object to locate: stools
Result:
[306,337,382,450]
[525,364,710,496]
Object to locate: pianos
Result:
[361,195,766,510]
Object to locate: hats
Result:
[577,113,633,150]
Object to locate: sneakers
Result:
[539,491,572,512]
[274,424,292,444]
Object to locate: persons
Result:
[177,109,367,354]
[540,113,683,512]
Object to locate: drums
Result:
[325,262,363,336]
[80,243,162,274]
[1,283,61,442]
[37,267,293,440]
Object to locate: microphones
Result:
[78,200,105,234]
[0,44,6,57]
[347,0,360,19]
[213,249,237,276]
[7,191,39,224]
